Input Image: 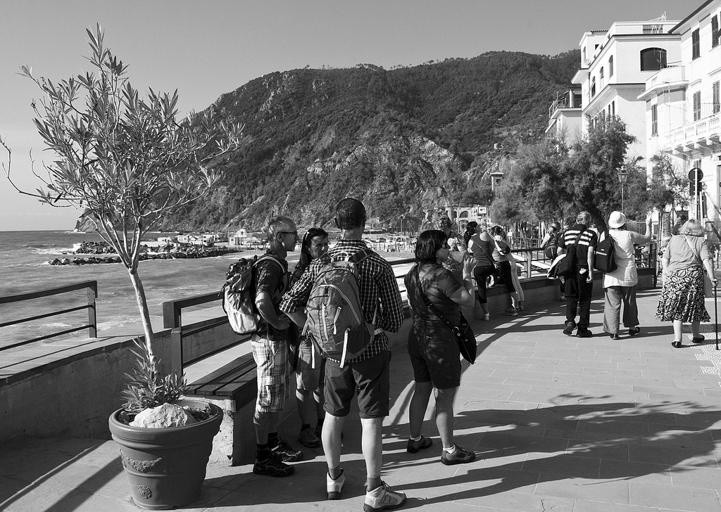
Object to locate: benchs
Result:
[0,281,172,443]
[162,292,258,467]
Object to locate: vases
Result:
[108,335,224,511]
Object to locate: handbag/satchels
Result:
[704,273,712,297]
[496,240,511,254]
[593,237,617,273]
[551,244,576,278]
[452,316,477,364]
[492,260,504,280]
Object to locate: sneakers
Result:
[253,426,476,512]
[563,322,704,348]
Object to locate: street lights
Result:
[617,165,629,213]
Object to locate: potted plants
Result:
[0,21,245,511]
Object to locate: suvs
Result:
[188,238,213,246]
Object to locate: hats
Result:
[608,211,626,228]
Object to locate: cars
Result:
[245,236,268,246]
[360,232,415,253]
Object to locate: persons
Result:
[464,221,495,320]
[437,213,466,251]
[540,210,652,340]
[404,230,477,466]
[654,219,717,348]
[279,197,406,512]
[490,226,525,317]
[290,227,343,448]
[252,218,302,476]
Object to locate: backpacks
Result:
[217,255,288,334]
[301,249,385,368]
